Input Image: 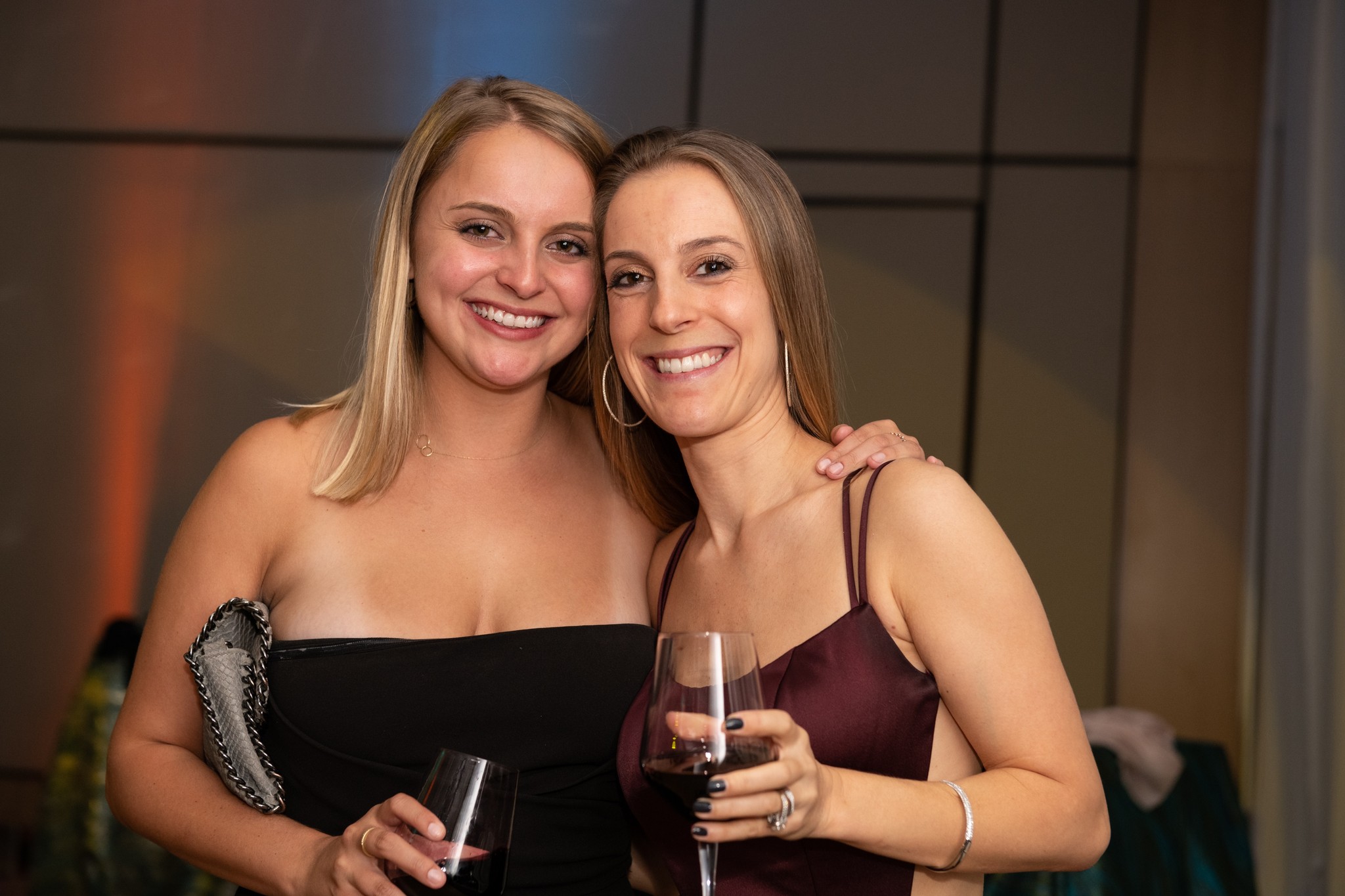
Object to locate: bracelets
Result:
[927,780,973,873]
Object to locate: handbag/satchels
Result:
[185,598,288,815]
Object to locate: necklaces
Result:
[416,380,553,461]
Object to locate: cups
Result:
[384,749,519,896]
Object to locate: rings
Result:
[361,827,377,859]
[782,788,795,818]
[767,787,788,832]
[106,79,949,894]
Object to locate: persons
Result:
[592,130,1112,895]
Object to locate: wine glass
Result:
[638,630,777,896]
